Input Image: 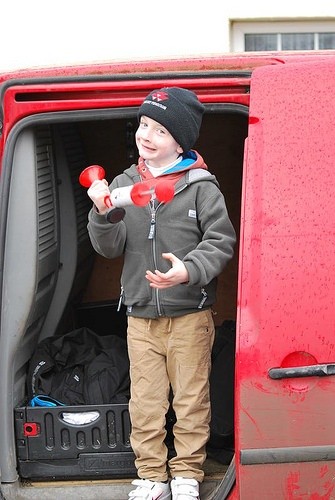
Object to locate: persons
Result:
[87,87,237,500]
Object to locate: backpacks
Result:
[24,326,131,402]
[205,321,236,466]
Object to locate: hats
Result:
[137,86,206,155]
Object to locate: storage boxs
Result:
[15,395,138,481]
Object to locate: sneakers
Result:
[128,475,200,500]
